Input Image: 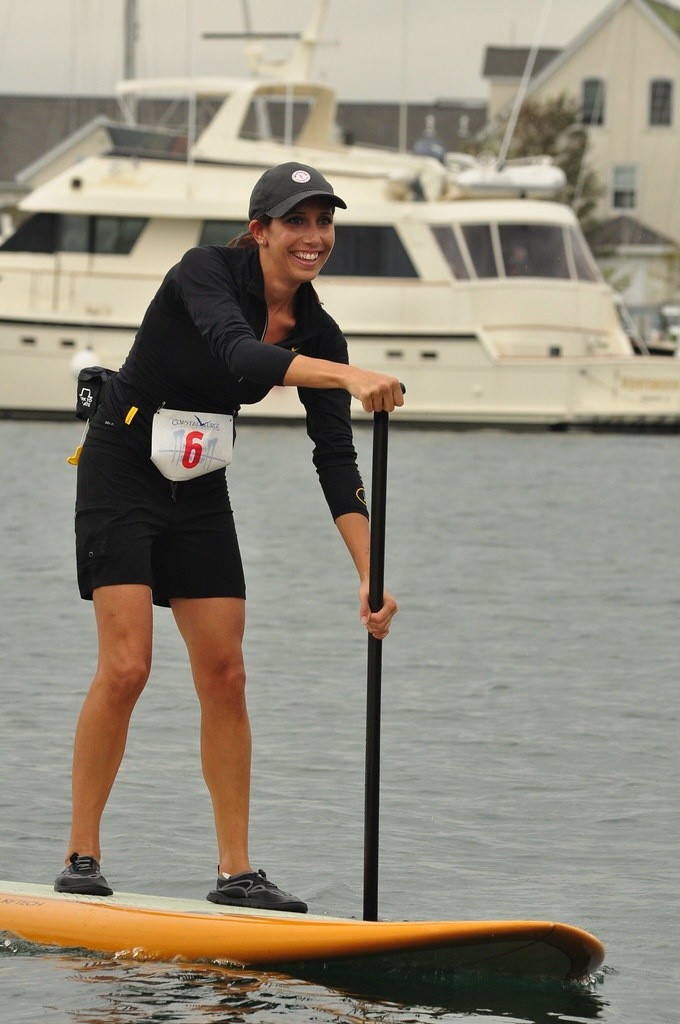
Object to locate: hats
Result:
[249,162,347,221]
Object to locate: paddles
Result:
[360,414,394,924]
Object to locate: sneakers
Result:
[206,865,308,912]
[54,852,113,896]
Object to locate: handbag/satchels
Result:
[75,366,117,421]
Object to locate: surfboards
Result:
[0,880,610,980]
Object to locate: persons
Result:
[56,161,405,913]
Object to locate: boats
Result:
[0,0,680,431]
